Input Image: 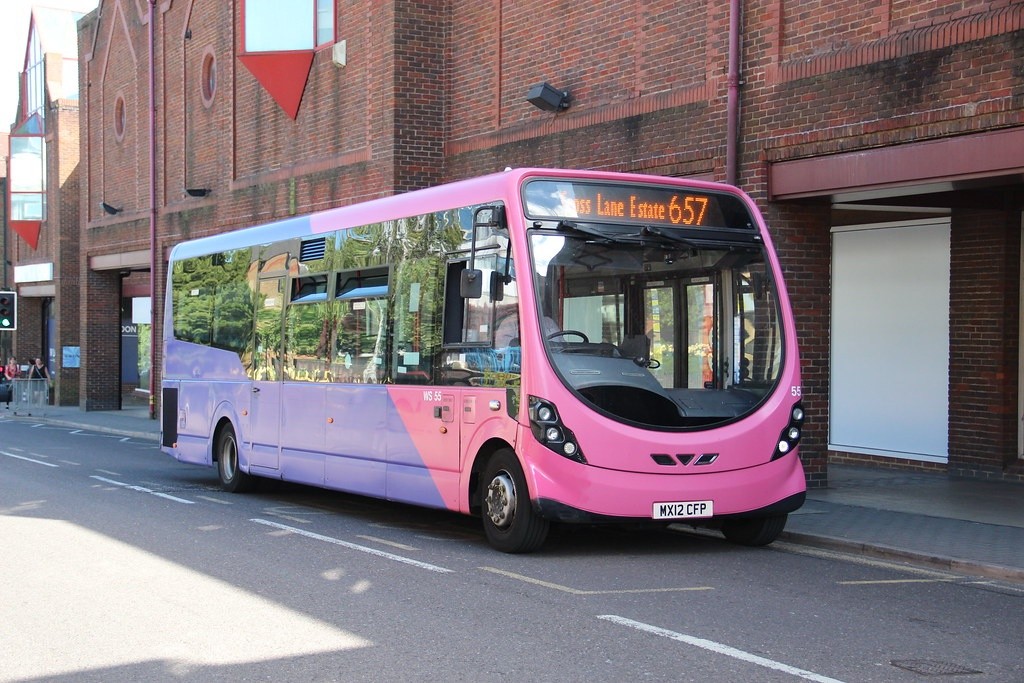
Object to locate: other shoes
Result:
[6,407,9,409]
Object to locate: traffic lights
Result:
[0,365,5,377]
[0,290,17,331]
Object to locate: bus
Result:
[159,167,808,551]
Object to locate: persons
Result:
[4,357,52,407]
[494,280,568,350]
